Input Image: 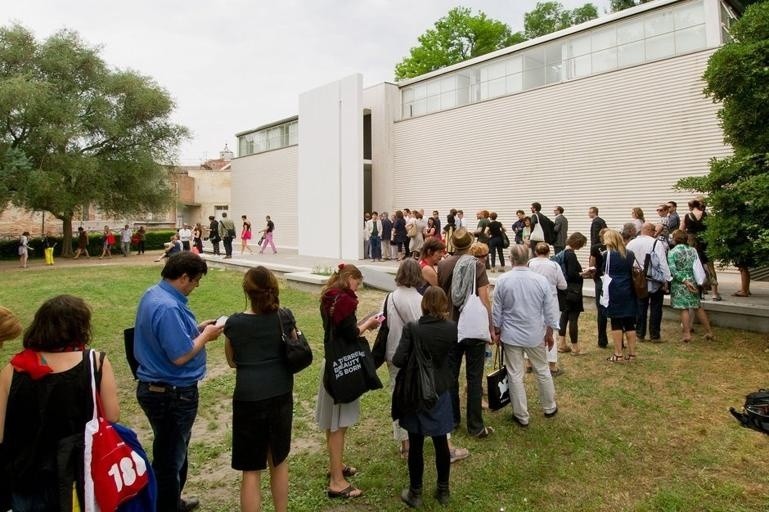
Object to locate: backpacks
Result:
[550,247,571,283]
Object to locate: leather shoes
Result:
[177,497,201,511]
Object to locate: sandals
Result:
[446,446,470,463]
[325,463,358,476]
[397,444,411,459]
[526,291,754,377]
[326,482,363,498]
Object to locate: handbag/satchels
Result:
[323,338,384,405]
[83,348,158,512]
[502,233,510,248]
[692,247,707,286]
[370,293,389,369]
[124,325,142,381]
[530,213,545,243]
[631,251,646,295]
[457,294,493,345]
[227,229,236,239]
[485,336,512,414]
[108,235,117,245]
[276,305,316,374]
[391,321,440,420]
[599,251,613,308]
[407,225,417,238]
[209,234,222,243]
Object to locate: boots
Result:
[432,483,450,504]
[400,487,423,508]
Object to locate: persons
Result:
[18,232,35,268]
[224,266,298,512]
[315,264,381,499]
[120,225,132,257]
[0,305,24,353]
[0,294,120,509]
[42,231,58,266]
[152,213,277,262]
[98,225,112,259]
[136,226,146,255]
[72,227,91,259]
[132,251,226,511]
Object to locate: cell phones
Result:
[212,315,230,327]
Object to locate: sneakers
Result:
[511,413,529,428]
[491,268,506,273]
[468,425,495,439]
[543,406,558,418]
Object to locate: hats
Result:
[450,228,474,249]
[471,242,490,258]
